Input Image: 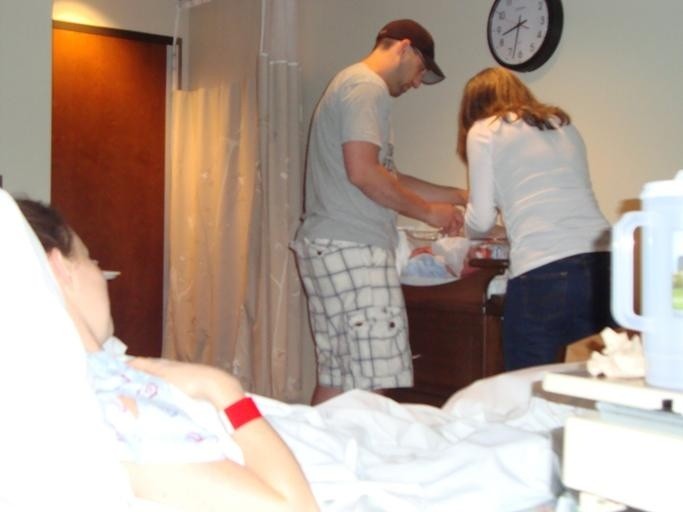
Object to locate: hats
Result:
[378,20,445,84]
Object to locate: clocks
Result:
[483,0,565,75]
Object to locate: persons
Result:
[289,19,468,406]
[15,197,320,511]
[456,66,613,370]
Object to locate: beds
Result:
[0,185,641,512]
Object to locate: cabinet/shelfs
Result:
[538,371,683,512]
[382,260,508,409]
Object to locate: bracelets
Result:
[218,397,261,435]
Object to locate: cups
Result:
[610,177,682,393]
